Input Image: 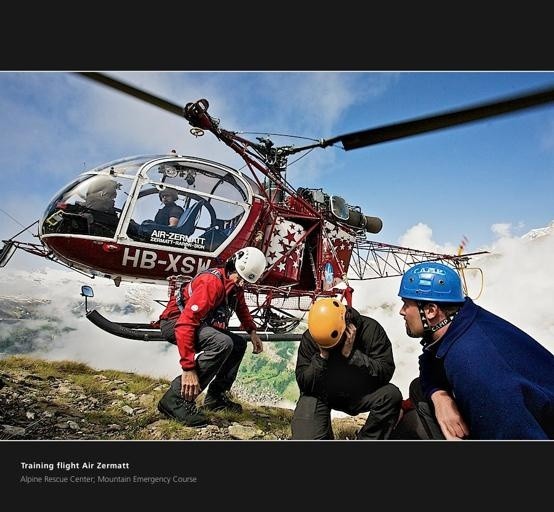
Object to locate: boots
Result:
[203,390,242,412]
[158,377,209,427]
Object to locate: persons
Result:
[394,260,554,440]
[155,245,268,430]
[287,297,404,439]
[140,187,185,228]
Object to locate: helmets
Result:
[159,188,178,201]
[232,247,267,284]
[398,263,466,302]
[307,298,347,349]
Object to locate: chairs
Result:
[138,200,233,252]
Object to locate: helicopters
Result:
[1,73,553,341]
